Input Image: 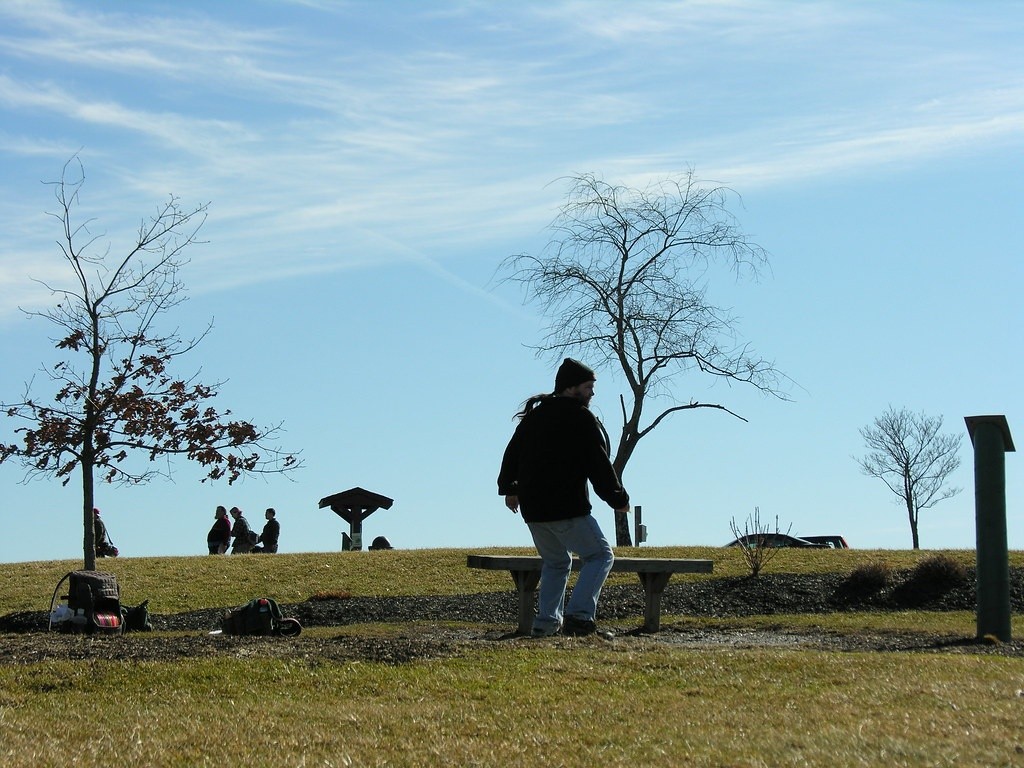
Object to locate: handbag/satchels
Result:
[103,546,118,557]
[119,600,153,636]
[247,531,259,545]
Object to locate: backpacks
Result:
[219,597,302,638]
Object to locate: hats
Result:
[555,358,596,391]
[93,508,100,514]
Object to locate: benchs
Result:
[466,554,714,632]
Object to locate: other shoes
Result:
[531,628,563,639]
[562,618,614,641]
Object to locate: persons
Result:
[258,508,279,553]
[497,358,630,641]
[231,507,253,554]
[207,506,231,554]
[94,508,106,558]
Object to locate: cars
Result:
[721,533,832,550]
